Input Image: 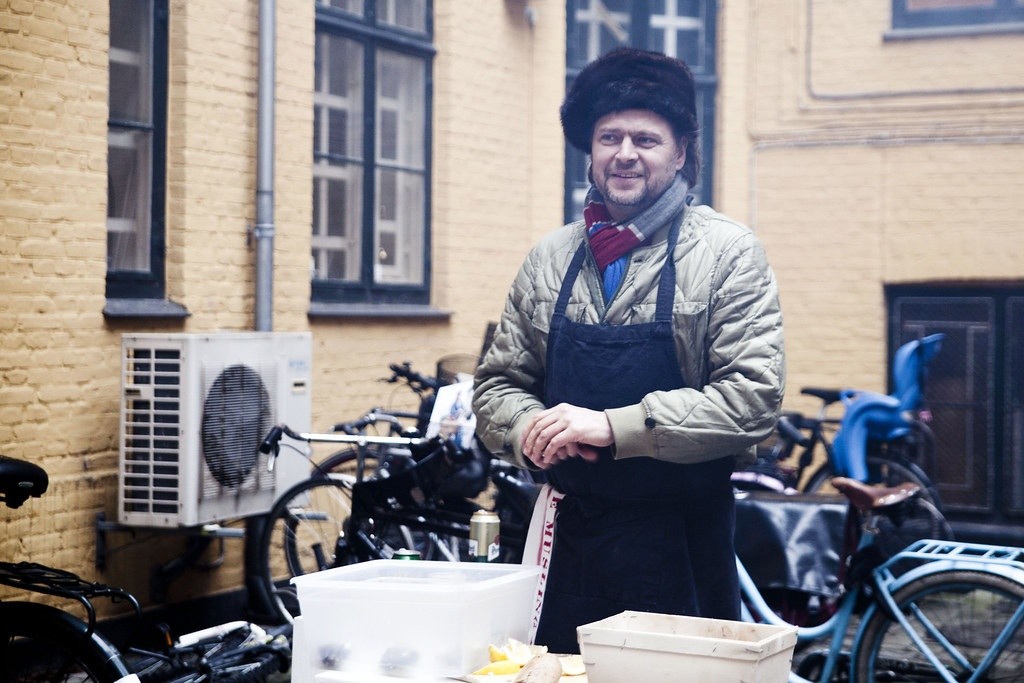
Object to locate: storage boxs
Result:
[291,616,528,683]
[577,610,798,683]
[290,558,544,634]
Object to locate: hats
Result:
[560,47,699,152]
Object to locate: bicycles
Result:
[246,322,1024,683]
[0,457,145,683]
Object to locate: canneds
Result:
[390,548,422,560]
[469,509,500,563]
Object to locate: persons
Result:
[471,46,785,654]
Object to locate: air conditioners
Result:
[118,330,310,527]
